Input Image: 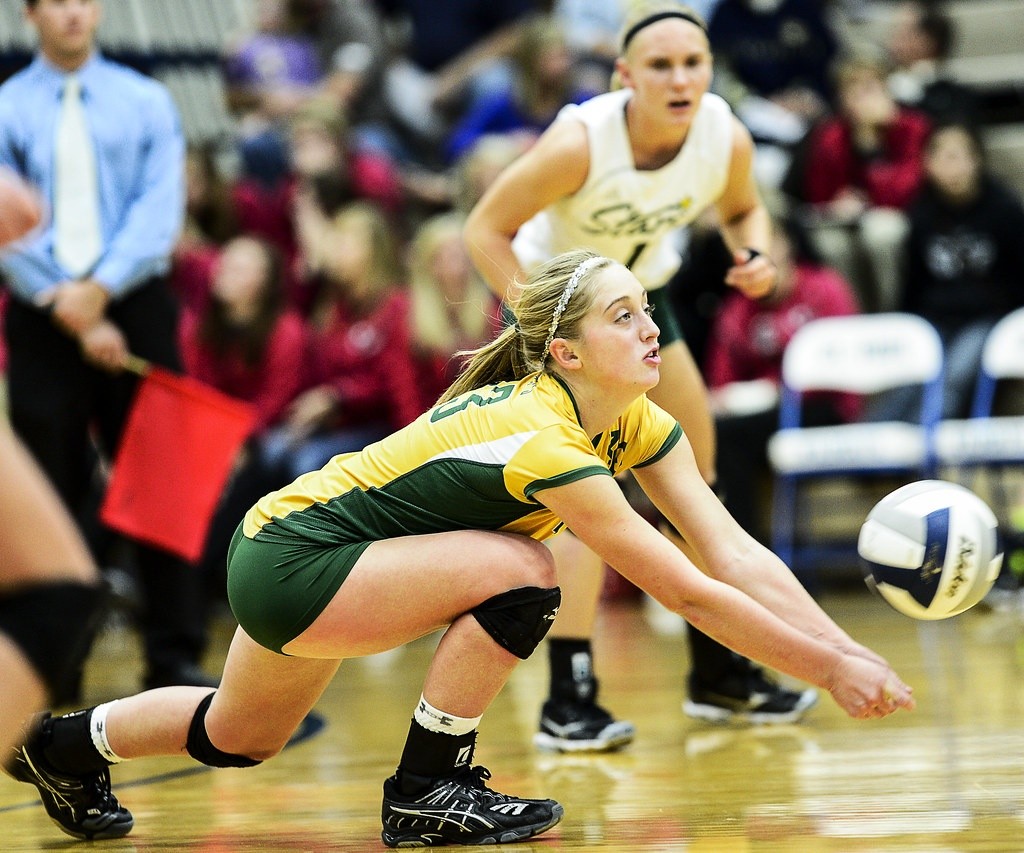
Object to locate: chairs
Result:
[767,305,1023,575]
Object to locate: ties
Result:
[53,80,107,280]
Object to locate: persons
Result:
[0,0,1024,853]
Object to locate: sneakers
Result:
[686,724,809,765]
[683,665,816,728]
[380,765,562,849]
[538,753,636,807]
[0,710,134,839]
[534,701,635,753]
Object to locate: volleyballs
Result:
[856,480,1007,623]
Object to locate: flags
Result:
[97,370,264,561]
